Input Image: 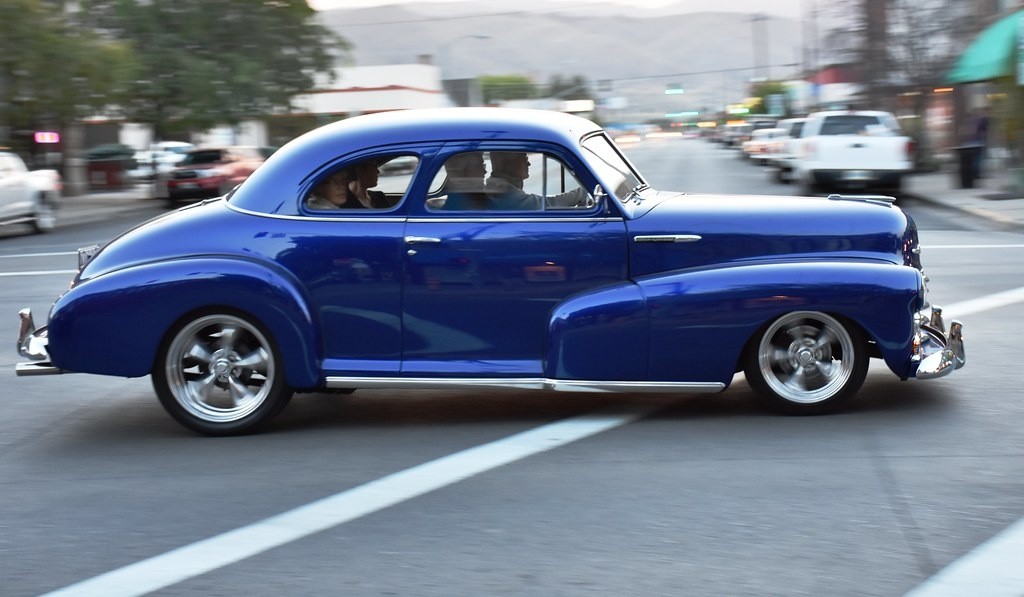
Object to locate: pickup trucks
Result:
[787,111,916,200]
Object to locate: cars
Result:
[14,106,966,437]
[0,153,66,232]
[168,146,267,204]
[716,119,786,166]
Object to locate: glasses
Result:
[513,157,528,163]
[479,163,486,169]
[323,178,348,187]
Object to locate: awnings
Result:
[940,7,1024,89]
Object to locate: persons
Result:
[442,150,586,210]
[307,156,391,210]
